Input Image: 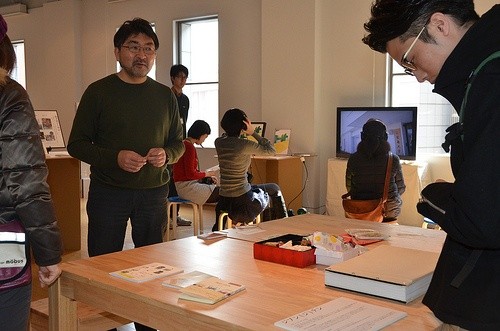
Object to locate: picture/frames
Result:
[242,120,267,139]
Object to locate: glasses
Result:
[118,45,157,55]
[175,75,187,80]
[401,11,448,76]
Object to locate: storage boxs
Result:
[313,246,358,266]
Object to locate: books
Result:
[161,270,219,289]
[324,244,442,303]
[108,262,183,283]
[177,277,244,305]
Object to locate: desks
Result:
[324,158,424,228]
[215,151,316,213]
[49,213,447,331]
[46,151,81,256]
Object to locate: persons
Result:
[0,14,62,331]
[213,108,288,232]
[174,120,227,232]
[166,65,190,229]
[362,0,500,331]
[67,18,186,331]
[345,118,406,223]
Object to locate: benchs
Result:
[30,297,134,331]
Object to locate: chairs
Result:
[341,197,397,225]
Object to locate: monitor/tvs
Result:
[337,106,417,160]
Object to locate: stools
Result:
[218,209,261,232]
[163,199,203,240]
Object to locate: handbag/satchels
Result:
[344,199,387,223]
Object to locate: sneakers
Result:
[170,218,174,229]
[172,215,192,226]
